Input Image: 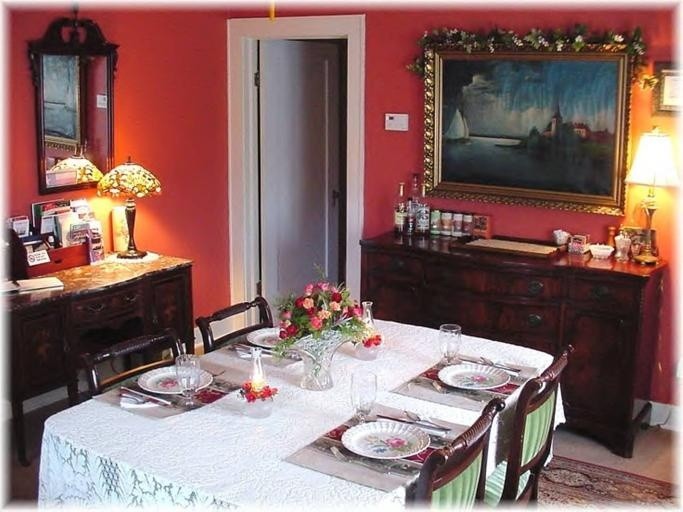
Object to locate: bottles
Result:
[356,301,379,360]
[617,233,631,262]
[245,346,272,419]
[404,173,420,236]
[415,181,430,238]
[392,181,408,235]
[614,230,625,257]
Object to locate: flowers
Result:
[273,280,383,362]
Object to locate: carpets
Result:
[537,454,681,510]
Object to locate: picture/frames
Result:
[654,61,681,114]
[423,39,634,218]
[41,54,86,153]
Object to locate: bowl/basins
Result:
[589,245,614,259]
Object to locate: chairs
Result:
[194,294,275,353]
[407,398,506,511]
[482,346,580,511]
[79,325,184,396]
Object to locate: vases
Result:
[282,332,353,390]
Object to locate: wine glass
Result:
[352,370,377,426]
[437,324,462,365]
[176,354,201,407]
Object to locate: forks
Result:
[119,393,149,405]
[431,378,484,403]
[329,445,390,479]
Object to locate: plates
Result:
[437,363,510,390]
[341,420,431,459]
[246,328,279,348]
[138,366,213,395]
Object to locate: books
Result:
[16,276,64,294]
[5,195,105,266]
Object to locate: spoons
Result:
[404,409,451,431]
[480,355,522,373]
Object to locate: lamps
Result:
[624,127,678,266]
[48,145,103,181]
[96,156,160,260]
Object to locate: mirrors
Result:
[26,4,120,195]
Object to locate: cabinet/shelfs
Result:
[9,273,81,467]
[360,227,455,330]
[64,259,147,330]
[455,244,565,367]
[565,252,667,459]
[121,258,190,355]
